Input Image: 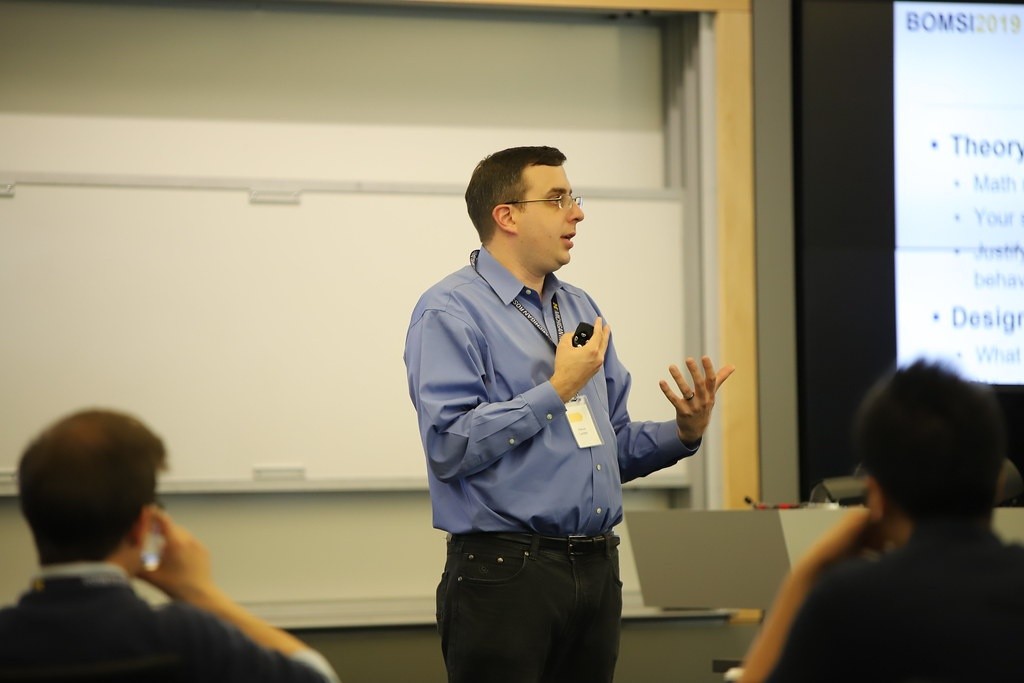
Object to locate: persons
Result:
[1,410,340,683]
[737,360,1024,683]
[403,146,737,683]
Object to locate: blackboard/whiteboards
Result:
[0,4,692,496]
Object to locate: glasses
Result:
[502,194,583,210]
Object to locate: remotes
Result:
[572,322,595,348]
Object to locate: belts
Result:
[485,531,621,558]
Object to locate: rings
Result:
[687,393,694,400]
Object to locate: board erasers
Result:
[252,466,309,481]
